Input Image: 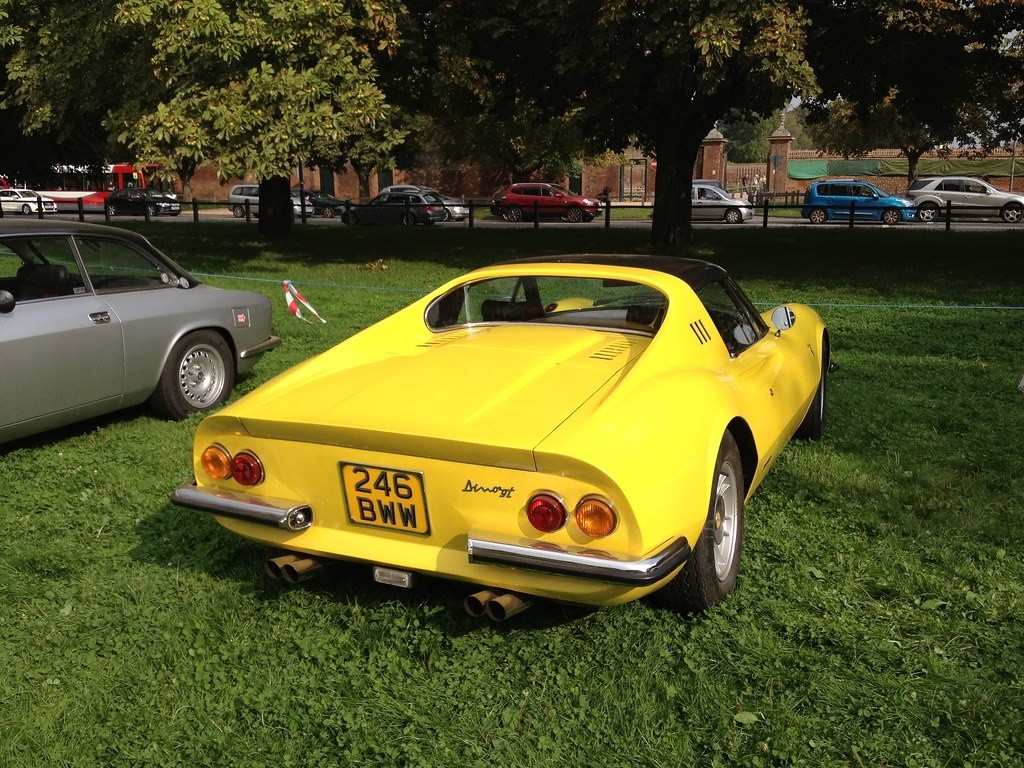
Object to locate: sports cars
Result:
[168,251,830,624]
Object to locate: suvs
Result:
[906,177,1024,223]
[800,179,916,225]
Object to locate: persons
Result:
[700,191,707,199]
[743,174,749,194]
[753,174,766,187]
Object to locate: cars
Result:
[379,184,470,223]
[0,216,285,447]
[290,188,353,217]
[489,182,602,222]
[341,192,446,227]
[0,188,57,215]
[650,179,755,224]
[102,188,181,217]
[227,184,314,217]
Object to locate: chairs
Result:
[482,300,532,319]
[15,263,66,302]
[627,306,664,328]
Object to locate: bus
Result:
[0,164,175,211]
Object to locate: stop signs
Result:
[652,159,658,170]
